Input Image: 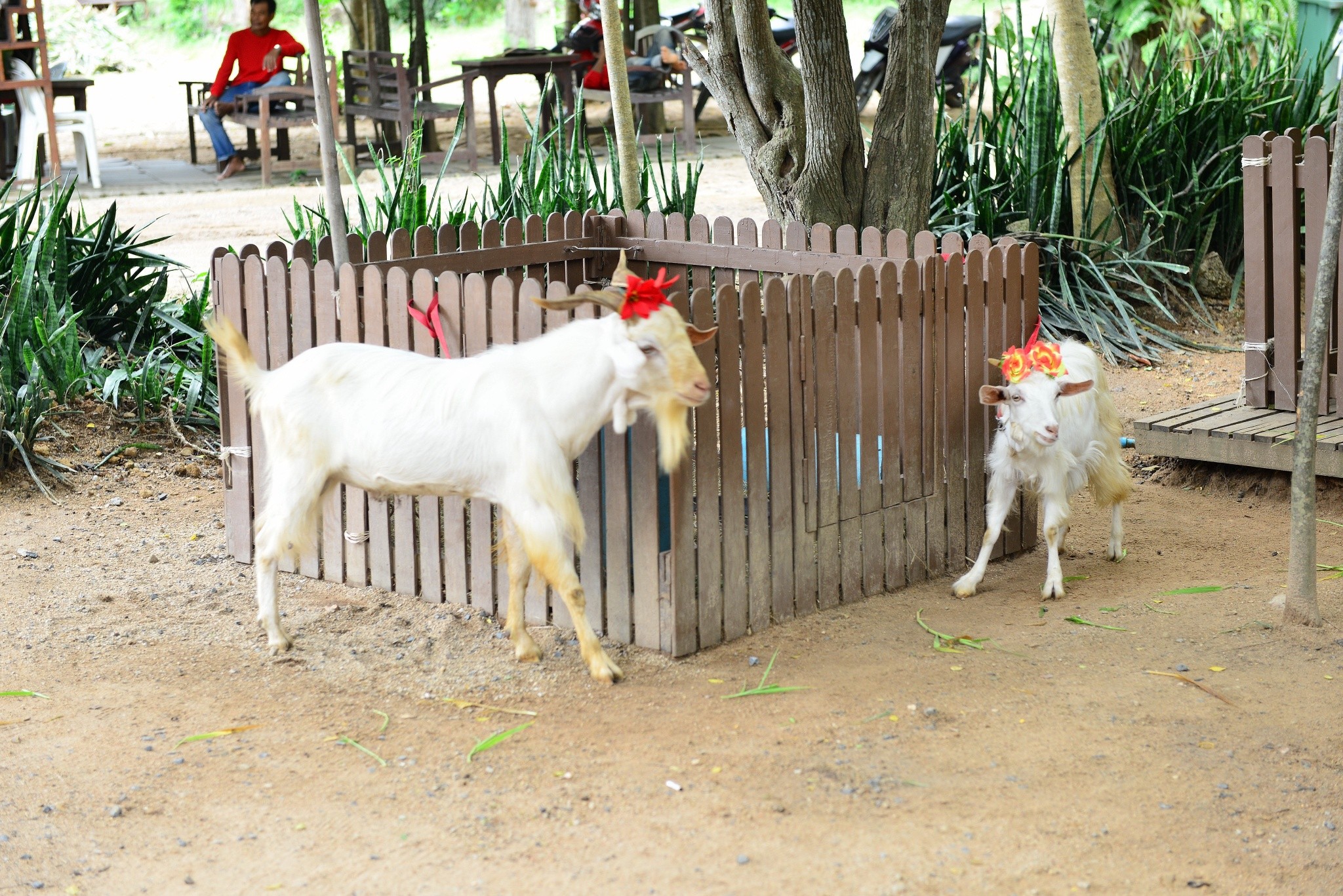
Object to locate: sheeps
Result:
[201,251,721,683]
[953,334,1135,602]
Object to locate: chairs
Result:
[4,50,102,192]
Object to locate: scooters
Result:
[853,6,992,114]
[691,7,802,122]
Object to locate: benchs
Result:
[340,49,465,192]
[178,52,340,188]
[572,24,698,156]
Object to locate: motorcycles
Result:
[568,0,709,90]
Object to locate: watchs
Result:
[274,44,282,52]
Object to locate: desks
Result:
[0,80,94,178]
[451,53,581,172]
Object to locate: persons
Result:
[580,34,688,93]
[198,0,305,183]
